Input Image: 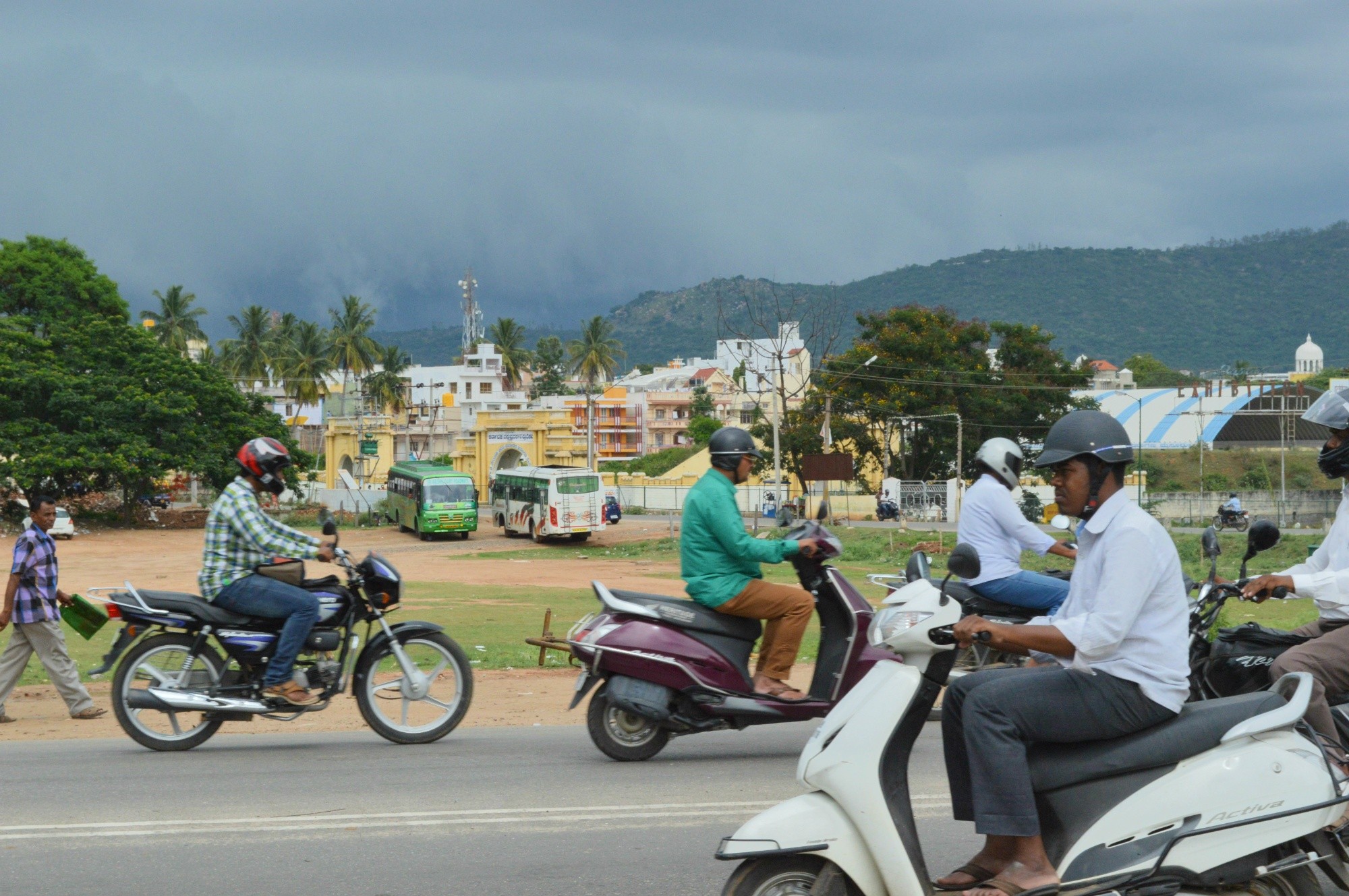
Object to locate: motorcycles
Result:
[1181,520,1349,767]
[876,497,900,522]
[714,543,1349,896]
[866,514,1078,721]
[1211,505,1249,532]
[85,509,475,751]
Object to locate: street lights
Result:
[587,368,641,469]
[1113,389,1142,506]
[820,354,879,525]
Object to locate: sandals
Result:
[263,679,320,706]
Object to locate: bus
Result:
[384,459,480,543]
[488,463,606,543]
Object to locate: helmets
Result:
[975,436,1024,490]
[708,427,764,463]
[235,437,292,496]
[1032,411,1134,468]
[1301,388,1349,479]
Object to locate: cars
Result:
[606,496,622,524]
[138,491,173,510]
[21,507,74,541]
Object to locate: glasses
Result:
[742,456,759,466]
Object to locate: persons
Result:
[931,410,1193,896]
[407,492,412,500]
[680,426,818,703]
[1223,491,1242,526]
[0,496,109,723]
[1204,380,1349,832]
[956,437,1072,667]
[876,487,894,517]
[198,437,337,706]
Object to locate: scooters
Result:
[564,498,904,762]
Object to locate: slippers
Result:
[978,877,1060,896]
[751,684,812,702]
[71,706,107,718]
[929,865,999,891]
[0,715,15,723]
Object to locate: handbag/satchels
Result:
[255,560,340,588]
